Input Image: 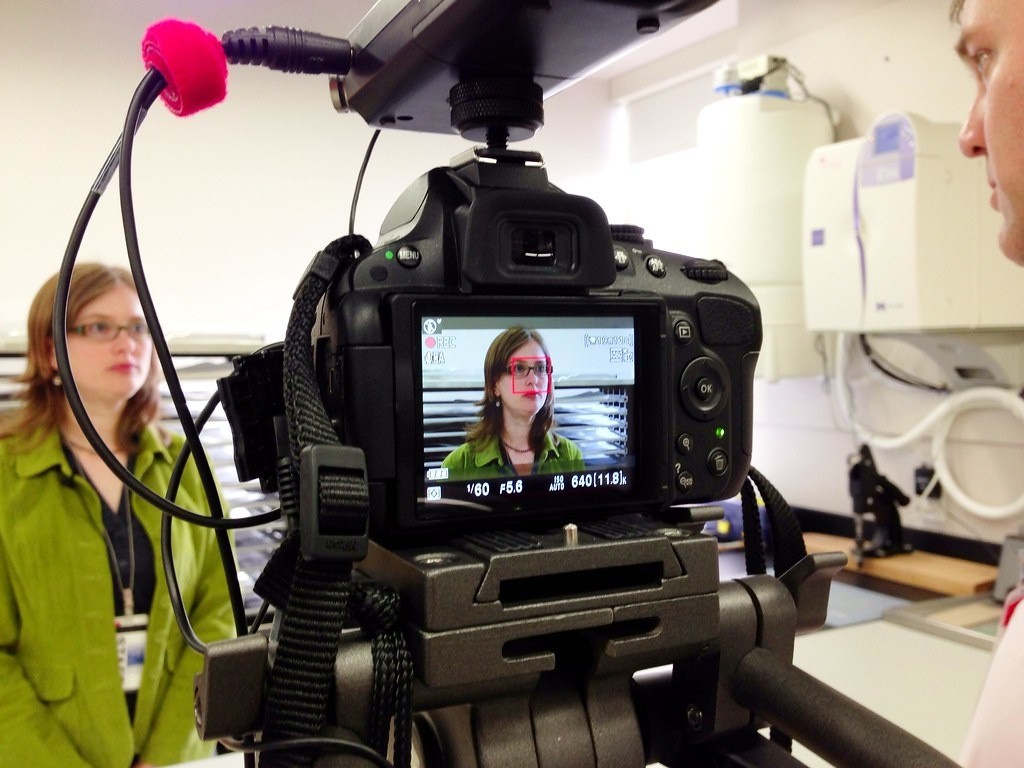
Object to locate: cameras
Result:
[308,146,765,543]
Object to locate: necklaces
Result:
[502,439,531,453]
[69,440,121,456]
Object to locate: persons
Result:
[953,0,1024,768]
[1,262,243,768]
[439,328,585,482]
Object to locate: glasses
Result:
[505,363,554,378]
[65,318,155,340]
